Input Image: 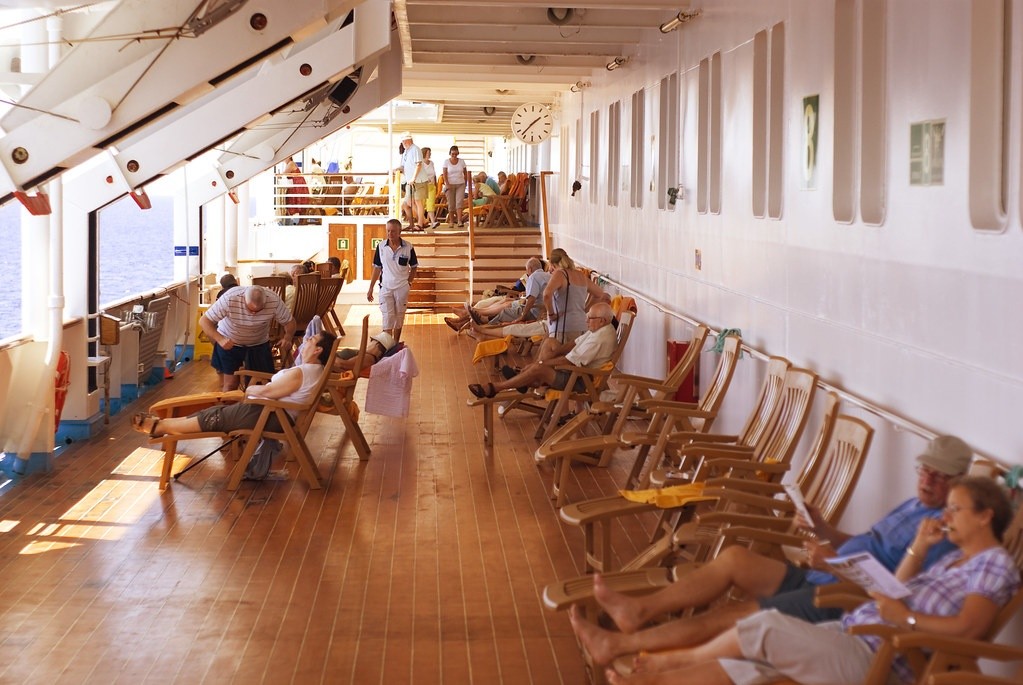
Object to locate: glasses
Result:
[916,463,961,485]
[587,316,607,322]
[944,505,990,513]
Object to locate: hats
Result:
[401,132,412,141]
[370,332,397,354]
[914,435,973,475]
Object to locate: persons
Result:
[199,257,393,392]
[604,476,1023,685]
[468,302,618,398]
[282,132,509,232]
[444,259,558,342]
[366,219,418,342]
[543,248,603,342]
[569,435,971,665]
[129,331,334,438]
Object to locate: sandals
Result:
[131,412,164,438]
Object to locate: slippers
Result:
[444,317,458,332]
[468,306,482,326]
[467,382,495,398]
[503,365,527,394]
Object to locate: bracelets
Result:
[549,312,554,316]
[906,547,925,562]
[410,281,413,285]
[521,312,526,318]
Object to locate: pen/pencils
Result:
[801,540,830,551]
[939,527,954,531]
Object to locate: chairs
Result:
[433,174,453,222]
[471,266,1022,685]
[300,176,395,225]
[149,264,371,493]
[464,172,528,228]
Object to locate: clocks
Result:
[510,102,553,144]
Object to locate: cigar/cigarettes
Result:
[370,300,372,303]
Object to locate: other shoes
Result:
[449,222,454,227]
[458,222,464,227]
[402,220,440,231]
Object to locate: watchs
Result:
[906,611,917,631]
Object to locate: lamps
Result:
[546,7,587,39]
[483,107,496,116]
[516,55,547,73]
[495,90,509,95]
